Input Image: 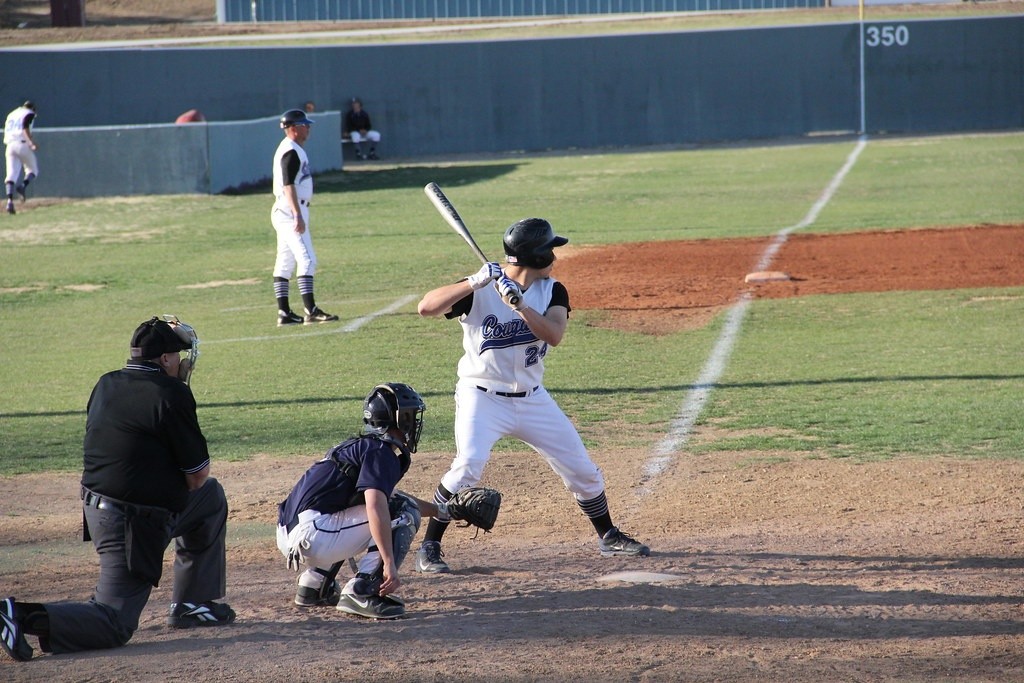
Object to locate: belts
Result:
[301,200,310,207]
[477,386,539,397]
[22,141,26,143]
[83,489,137,515]
[286,517,299,533]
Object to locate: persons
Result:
[277,383,501,618]
[416,217,651,573]
[304,102,315,113]
[343,97,381,160]
[0,314,237,661]
[271,109,338,327]
[3,100,39,214]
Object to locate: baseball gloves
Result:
[448,485,501,530]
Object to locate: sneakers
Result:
[167,601,236,627]
[304,306,338,324]
[336,577,406,618]
[597,526,649,555]
[295,569,341,606]
[277,310,304,326]
[0,597,33,660]
[416,541,450,574]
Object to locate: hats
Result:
[129,316,192,360]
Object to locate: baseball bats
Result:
[424,181,519,304]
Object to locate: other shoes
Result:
[16,187,25,202]
[6,203,15,214]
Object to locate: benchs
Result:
[341,135,372,145]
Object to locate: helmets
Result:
[503,218,568,269]
[280,109,314,128]
[24,101,33,108]
[361,382,419,434]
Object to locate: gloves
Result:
[493,275,528,312]
[287,531,310,572]
[468,262,502,290]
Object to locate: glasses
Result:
[295,124,309,127]
